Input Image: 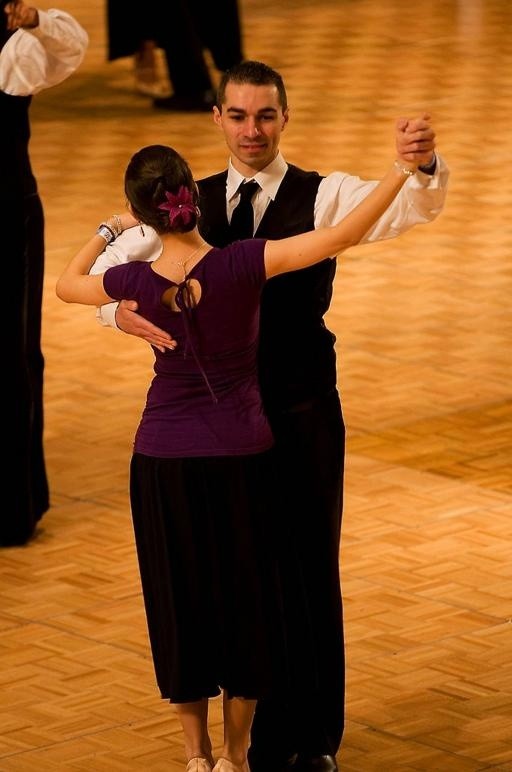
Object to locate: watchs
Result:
[1,0,88,549]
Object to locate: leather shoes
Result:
[130,69,219,112]
[183,742,342,772]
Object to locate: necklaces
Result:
[159,241,207,280]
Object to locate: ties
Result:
[226,179,262,243]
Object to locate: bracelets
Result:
[102,215,122,238]
[96,223,114,244]
[391,159,415,181]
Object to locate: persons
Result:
[128,41,174,99]
[105,0,246,116]
[55,111,429,770]
[86,62,450,771]
